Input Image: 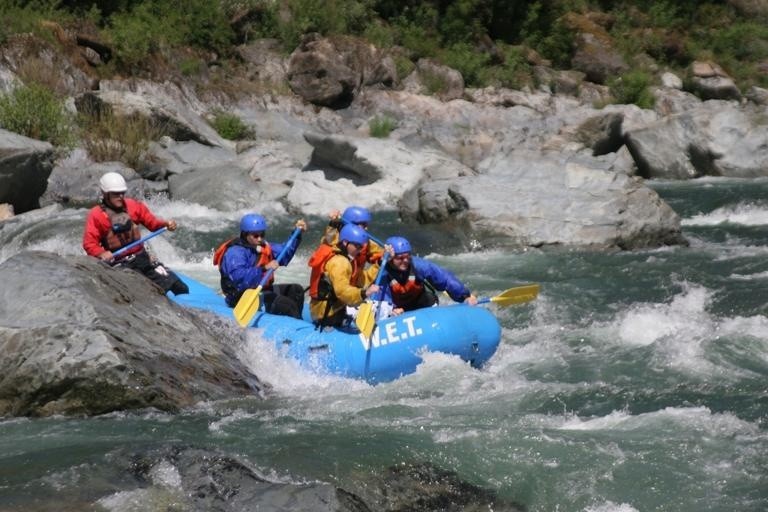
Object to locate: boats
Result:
[166,272,501,387]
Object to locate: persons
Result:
[82,171,190,297]
[215,212,304,322]
[368,236,478,319]
[319,206,395,287]
[307,222,395,331]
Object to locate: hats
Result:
[341,206,372,228]
[385,236,413,256]
[99,172,128,195]
[338,225,369,247]
[239,212,266,234]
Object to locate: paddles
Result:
[478,285,538,305]
[356,252,388,340]
[233,219,304,328]
